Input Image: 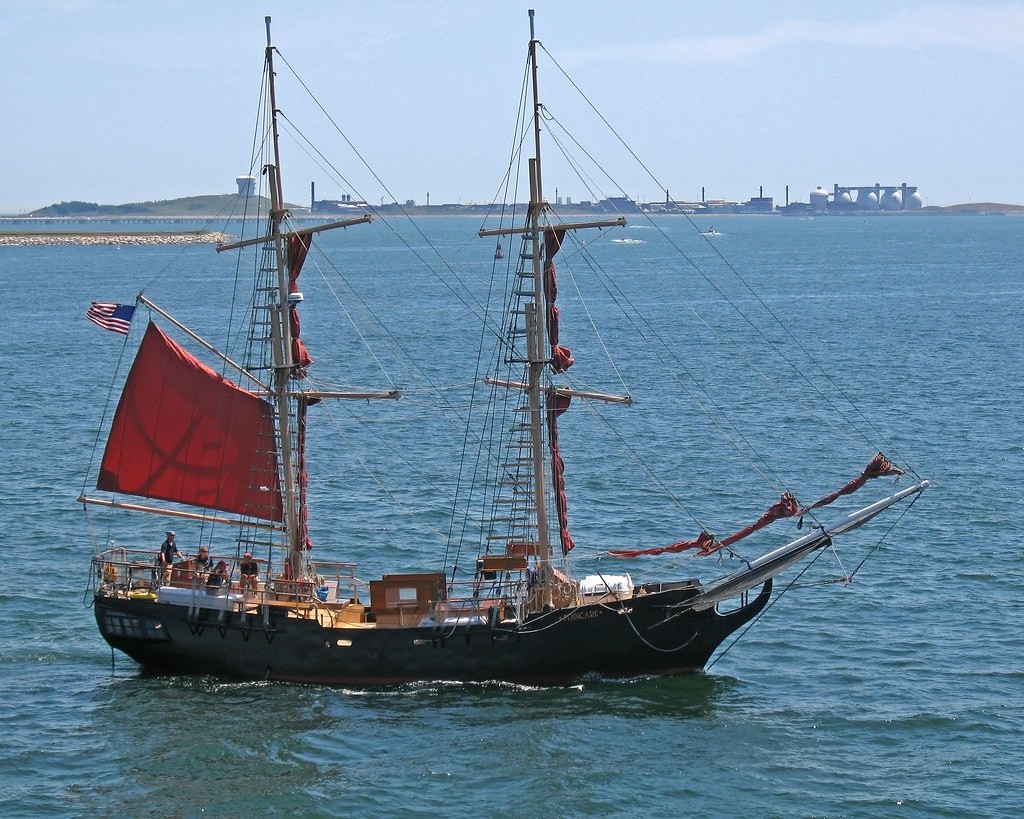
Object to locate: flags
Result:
[86,301,136,334]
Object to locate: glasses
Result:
[169,535,175,536]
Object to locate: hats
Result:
[217,560,230,567]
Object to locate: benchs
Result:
[234,598,314,618]
[229,581,266,598]
[154,586,244,610]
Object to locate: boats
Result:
[75,7,931,690]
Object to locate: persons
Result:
[192,546,215,584]
[159,531,185,587]
[238,553,260,599]
[205,561,232,595]
